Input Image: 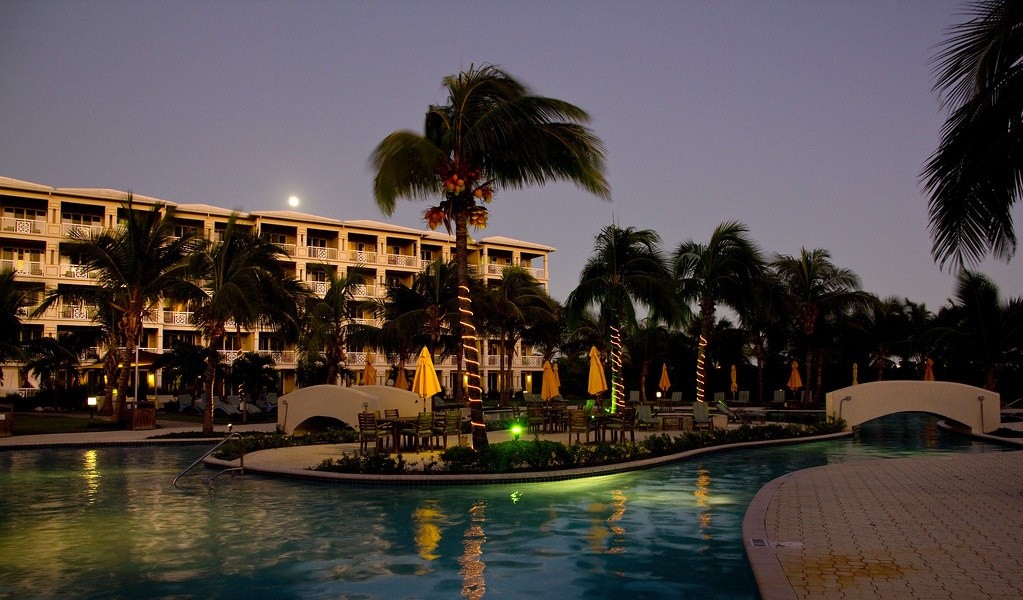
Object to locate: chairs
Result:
[358,409,462,454]
[628,390,812,431]
[179,392,279,415]
[513,399,636,446]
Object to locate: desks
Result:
[376,417,418,454]
[663,416,683,430]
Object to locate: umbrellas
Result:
[787,360,802,402]
[659,363,671,399]
[924,358,934,381]
[731,365,737,401]
[588,345,608,409]
[364,350,377,385]
[394,361,409,390]
[852,363,858,386]
[412,345,442,415]
[541,361,561,400]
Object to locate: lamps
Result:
[227,424,233,433]
[88,397,97,419]
[511,426,521,441]
